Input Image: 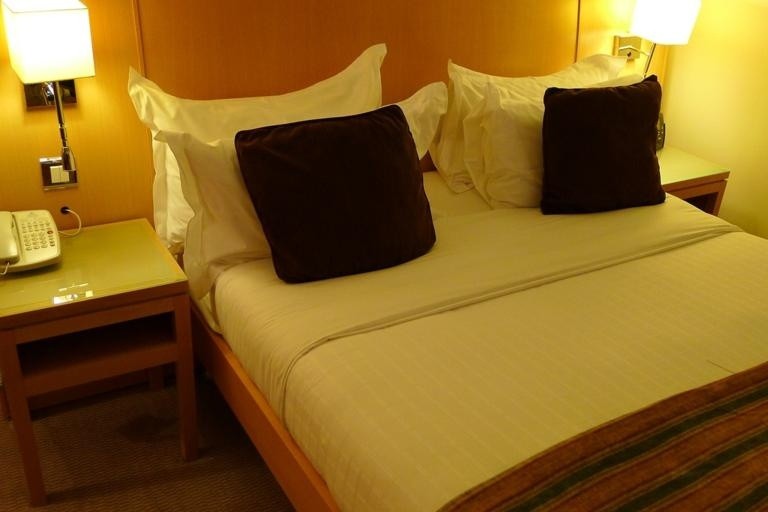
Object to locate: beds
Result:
[175,190,768,512]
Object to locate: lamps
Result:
[1,0,95,171]
[614,0,701,78]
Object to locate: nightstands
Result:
[0,219,196,507]
[656,146,730,216]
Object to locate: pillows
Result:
[234,104,436,284]
[127,43,386,258]
[431,54,621,193]
[541,74,667,214]
[155,81,448,299]
[464,76,648,208]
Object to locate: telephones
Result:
[0,209,62,274]
